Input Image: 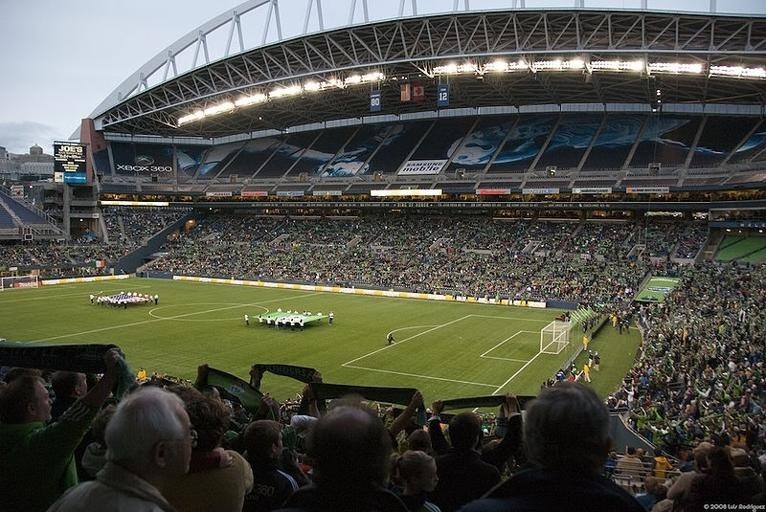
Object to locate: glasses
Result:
[161,430,199,447]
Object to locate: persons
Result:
[0,192,766,512]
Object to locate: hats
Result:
[245,420,284,454]
